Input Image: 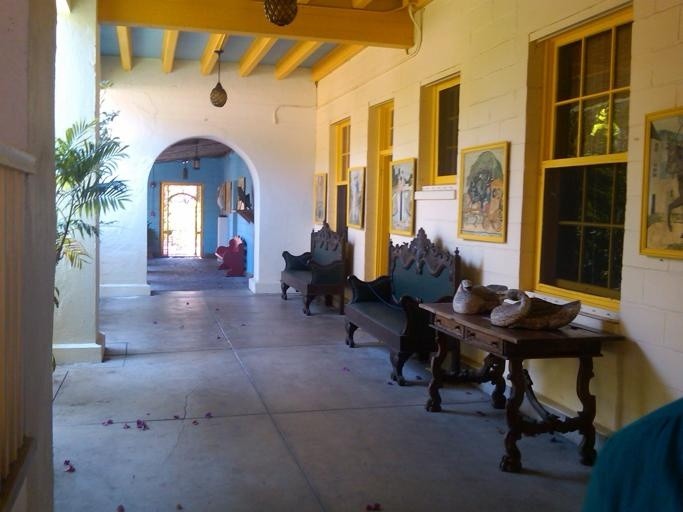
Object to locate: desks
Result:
[418,303,626,473]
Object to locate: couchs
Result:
[280,223,347,316]
[344,228,460,386]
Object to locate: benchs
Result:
[214,235,245,277]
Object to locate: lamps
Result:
[210,50,228,107]
[264,0,297,27]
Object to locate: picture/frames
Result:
[313,172,327,224]
[389,158,417,236]
[457,140,508,242]
[346,167,365,228]
[639,106,683,259]
